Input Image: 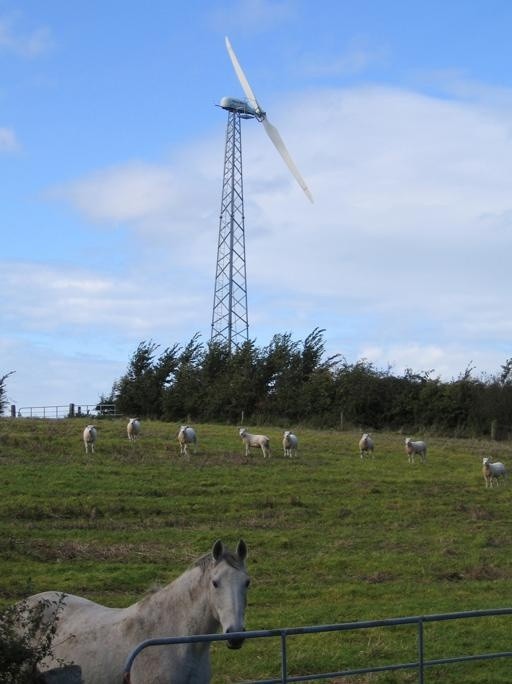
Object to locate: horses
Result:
[0,539,250,684]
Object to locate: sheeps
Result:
[83,425,97,454]
[177,425,197,456]
[482,457,506,488]
[359,433,375,459]
[239,427,272,459]
[405,438,426,464]
[126,417,141,443]
[282,430,297,457]
